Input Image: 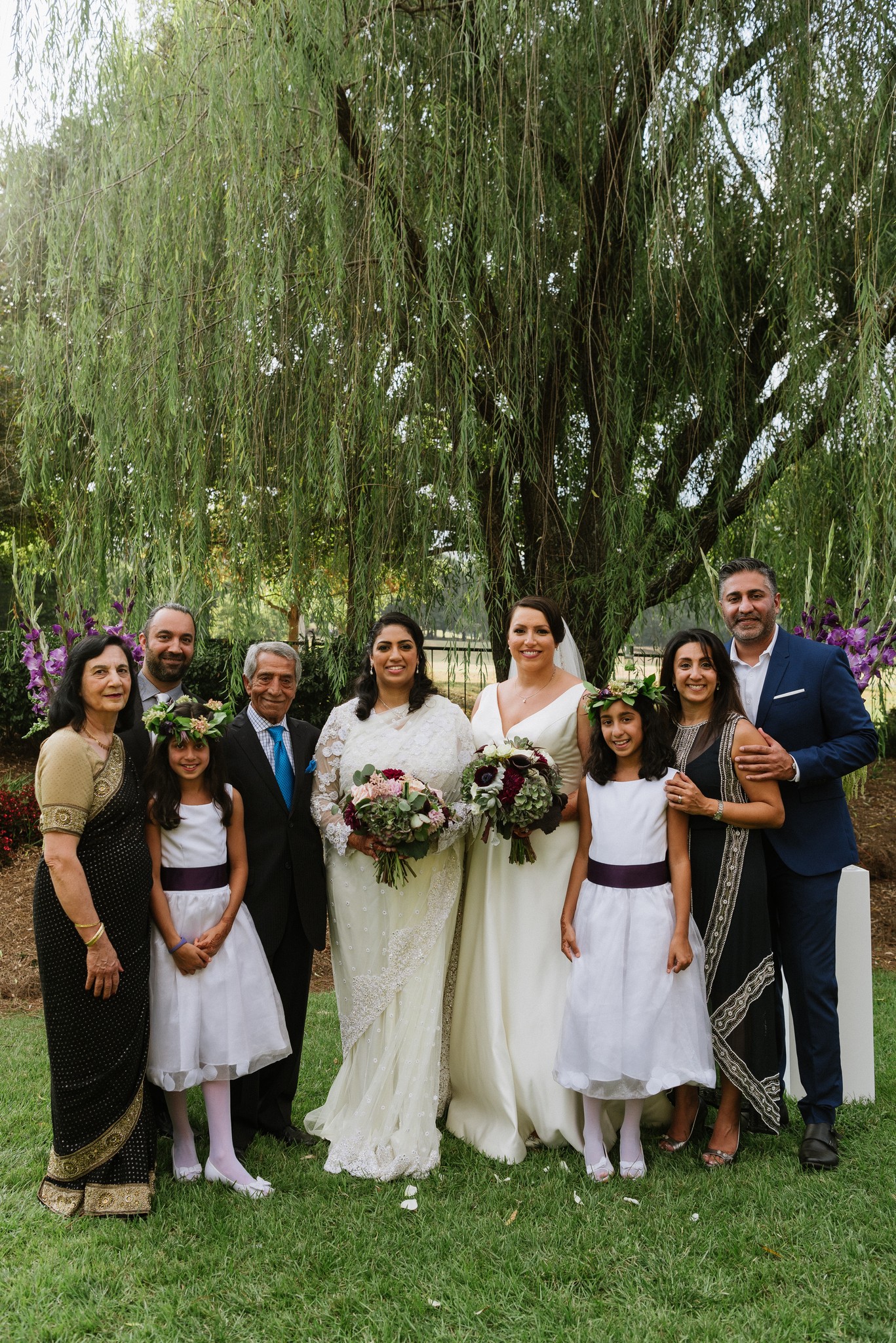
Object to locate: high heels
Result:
[659,1095,708,1153]
[701,1115,740,1167]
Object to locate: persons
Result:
[32,633,155,1221]
[140,691,294,1200]
[717,556,879,1171]
[210,639,328,1169]
[657,626,786,1164]
[444,597,671,1159]
[305,612,480,1185]
[552,669,716,1182]
[139,607,208,1141]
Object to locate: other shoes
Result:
[204,1159,275,1200]
[171,1143,202,1185]
[525,1130,543,1148]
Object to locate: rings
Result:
[370,843,374,850]
[525,827,528,832]
[678,796,682,804]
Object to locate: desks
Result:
[776,859,878,1111]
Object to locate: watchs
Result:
[792,762,797,775]
[710,799,723,821]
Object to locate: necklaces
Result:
[514,664,556,703]
[378,695,412,719]
[83,724,117,749]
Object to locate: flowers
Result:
[329,761,464,893]
[456,733,569,868]
[579,672,677,729]
[15,573,147,742]
[789,576,896,806]
[141,692,236,749]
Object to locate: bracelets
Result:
[88,927,105,946]
[75,920,100,928]
[168,937,187,954]
[85,922,104,945]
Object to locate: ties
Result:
[152,693,170,748]
[268,726,294,812]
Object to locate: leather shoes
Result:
[775,1100,791,1129]
[268,1125,317,1146]
[799,1122,844,1169]
[231,1125,257,1160]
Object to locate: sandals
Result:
[584,1141,615,1185]
[619,1139,647,1181]
